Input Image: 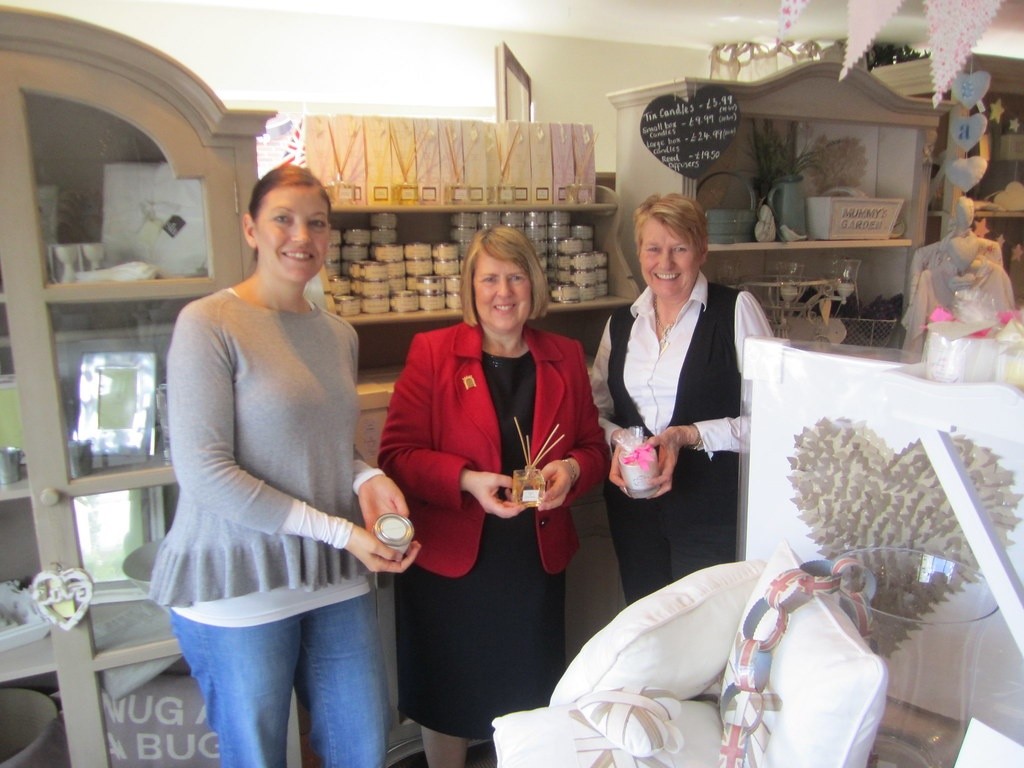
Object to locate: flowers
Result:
[742,114,867,239]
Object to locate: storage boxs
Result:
[298,115,597,204]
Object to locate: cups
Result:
[156,384,171,460]
[836,546,999,768]
[0,447,23,485]
[66,438,93,478]
[618,447,661,499]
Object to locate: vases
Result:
[832,547,1004,768]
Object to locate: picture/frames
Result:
[71,483,169,604]
[737,337,1024,768]
[76,350,155,454]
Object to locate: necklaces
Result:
[653,296,667,329]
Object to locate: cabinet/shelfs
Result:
[0,3,284,768]
[316,202,645,405]
[605,51,1023,344]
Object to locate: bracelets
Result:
[689,440,702,450]
[563,460,577,483]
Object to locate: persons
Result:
[148,167,421,768]
[589,192,773,604]
[377,226,612,768]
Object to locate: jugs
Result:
[768,174,806,240]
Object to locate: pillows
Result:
[492,699,723,768]
[102,677,224,768]
[721,537,890,768]
[550,560,767,706]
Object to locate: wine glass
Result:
[56,241,102,284]
